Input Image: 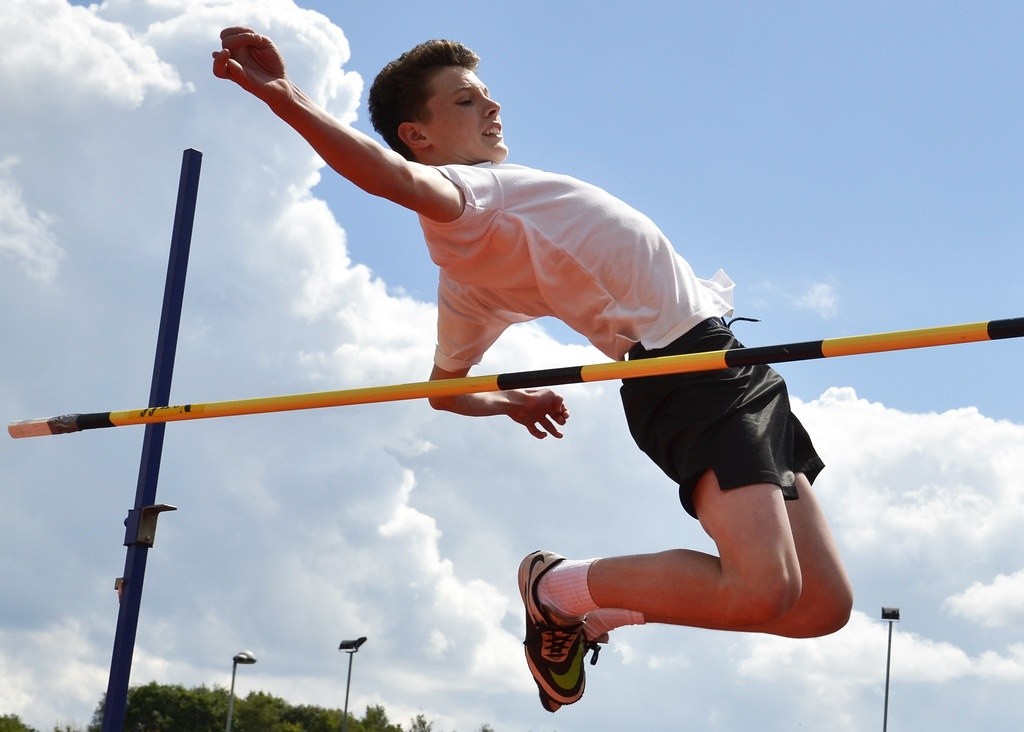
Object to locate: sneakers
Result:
[539,688,562,713]
[517,550,585,703]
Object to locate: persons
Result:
[211,25,857,714]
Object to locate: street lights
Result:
[876,605,902,731]
[220,651,259,732]
[334,636,371,732]
